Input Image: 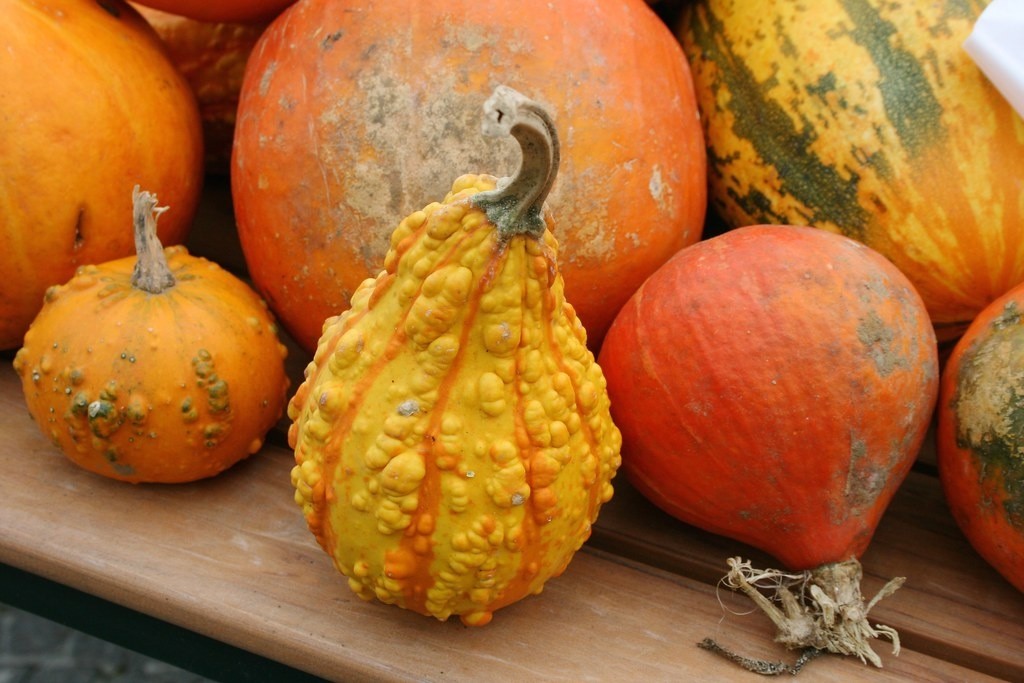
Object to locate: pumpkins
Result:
[0,0,1024,676]
